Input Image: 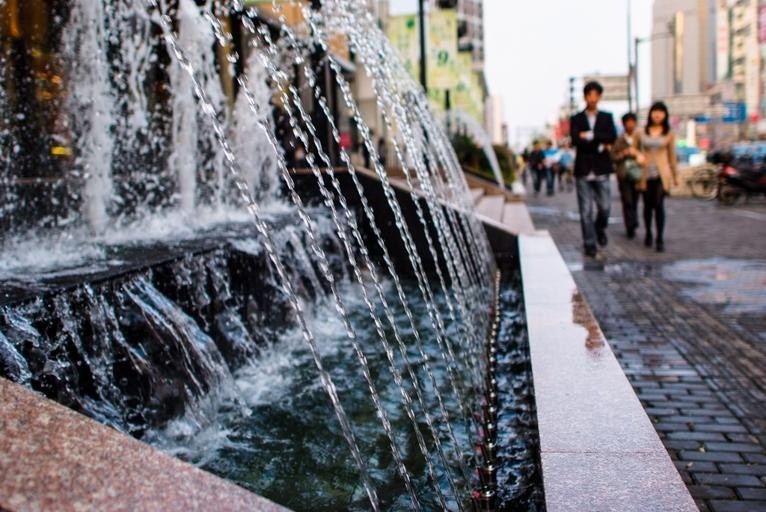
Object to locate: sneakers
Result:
[594,222,607,247]
[655,236,663,251]
[645,231,653,246]
[586,247,596,259]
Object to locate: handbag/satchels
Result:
[624,159,641,182]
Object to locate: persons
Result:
[517,140,576,197]
[568,81,617,258]
[360,129,388,171]
[630,101,678,251]
[608,113,648,239]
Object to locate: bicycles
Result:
[684,163,723,202]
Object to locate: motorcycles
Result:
[707,148,766,204]
